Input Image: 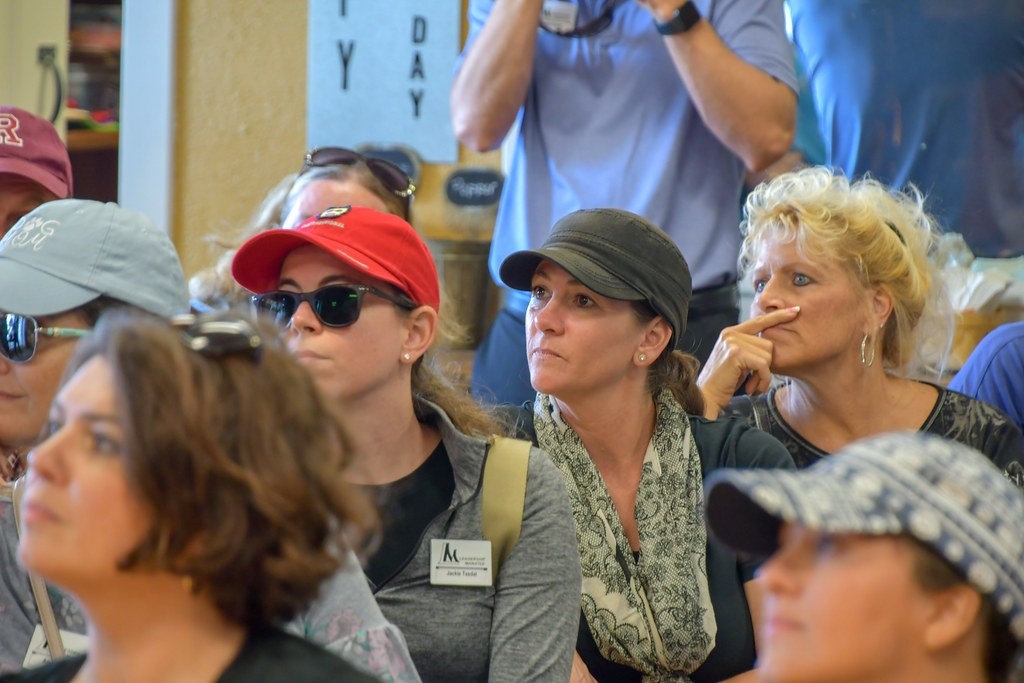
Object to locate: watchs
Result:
[655,1,700,34]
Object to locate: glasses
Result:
[0,313,94,363]
[251,279,417,332]
[294,146,415,209]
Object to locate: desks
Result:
[65,125,120,202]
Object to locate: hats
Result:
[0,105,74,199]
[0,199,191,326]
[500,207,691,352]
[231,204,440,317]
[704,431,1023,646]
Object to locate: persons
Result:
[947,320,1024,439]
[0,106,73,241]
[706,429,1024,683]
[451,0,799,407]
[469,207,798,683]
[191,147,417,316]
[0,307,384,683]
[0,197,420,683]
[230,202,581,683]
[696,169,1024,492]
